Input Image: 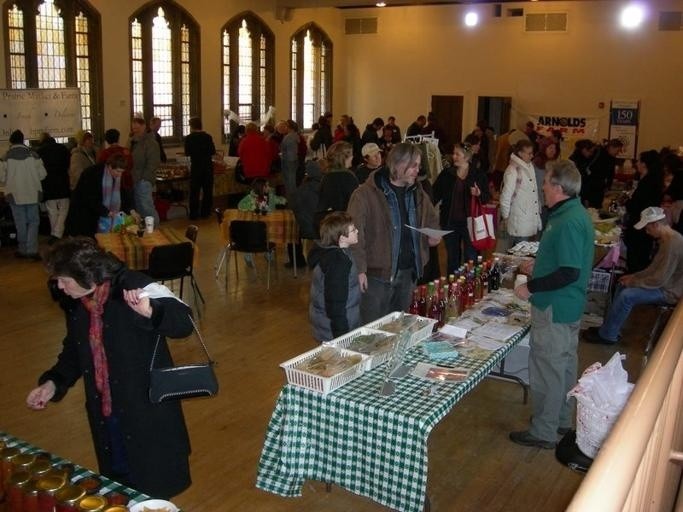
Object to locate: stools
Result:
[158,188,189,217]
[639,300,677,356]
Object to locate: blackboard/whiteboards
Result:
[0,88,82,141]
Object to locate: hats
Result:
[361,142,383,157]
[633,207,666,230]
[9,129,24,144]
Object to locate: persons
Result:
[227,112,444,270]
[184,117,218,222]
[458,120,626,216]
[347,143,443,324]
[429,142,490,275]
[534,138,560,200]
[26,235,194,498]
[500,139,543,244]
[509,159,594,448]
[0,113,177,271]
[583,207,683,343]
[615,143,683,271]
[309,211,363,342]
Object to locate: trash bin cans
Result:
[576,382,635,459]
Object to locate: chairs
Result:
[224,219,279,296]
[213,207,240,281]
[136,241,194,301]
[170,224,206,305]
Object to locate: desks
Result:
[152,156,262,203]
[491,211,625,324]
[217,207,300,279]
[254,251,536,512]
[93,231,200,285]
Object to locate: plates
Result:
[130,499,179,512]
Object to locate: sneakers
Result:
[27,255,42,263]
[510,432,554,449]
[15,252,26,259]
[583,326,623,344]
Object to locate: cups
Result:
[145,216,154,234]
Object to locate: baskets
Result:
[329,326,401,371]
[363,310,439,350]
[280,342,369,395]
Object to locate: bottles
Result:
[120,215,127,235]
[409,256,500,332]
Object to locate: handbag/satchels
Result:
[465,213,496,251]
[149,363,218,402]
